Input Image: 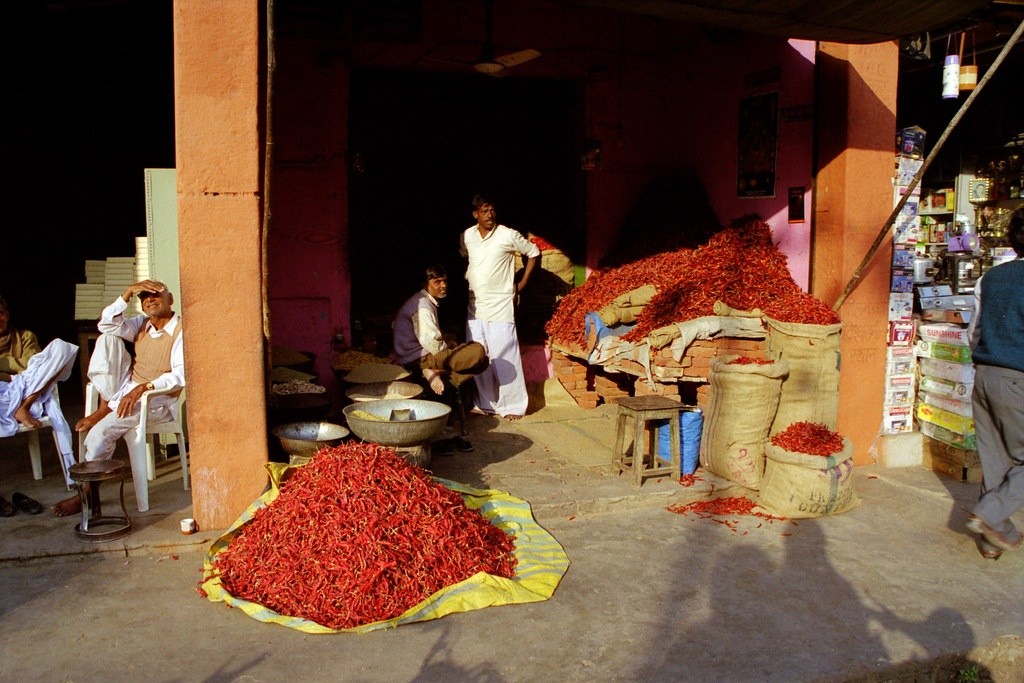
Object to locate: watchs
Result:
[146,381,154,390]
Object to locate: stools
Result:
[68,460,139,544]
[612,395,694,487]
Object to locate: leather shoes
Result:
[964,514,1021,551]
[982,528,1024,560]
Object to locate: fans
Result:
[424,0,543,76]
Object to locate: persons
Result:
[393,263,484,423]
[964,204,1024,561]
[464,192,541,419]
[0,296,79,485]
[50,280,185,517]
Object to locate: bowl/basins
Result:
[341,399,452,448]
[344,372,413,386]
[345,381,424,403]
[330,365,351,381]
[272,349,326,408]
[272,422,349,457]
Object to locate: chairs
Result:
[1,384,72,486]
[81,379,190,512]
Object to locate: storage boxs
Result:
[881,124,983,484]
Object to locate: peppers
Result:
[170,440,519,629]
[530,219,841,366]
[657,418,879,538]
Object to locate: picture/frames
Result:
[969,178,990,201]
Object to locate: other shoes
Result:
[0,499,15,517]
[439,439,453,455]
[451,435,474,452]
[12,491,43,516]
